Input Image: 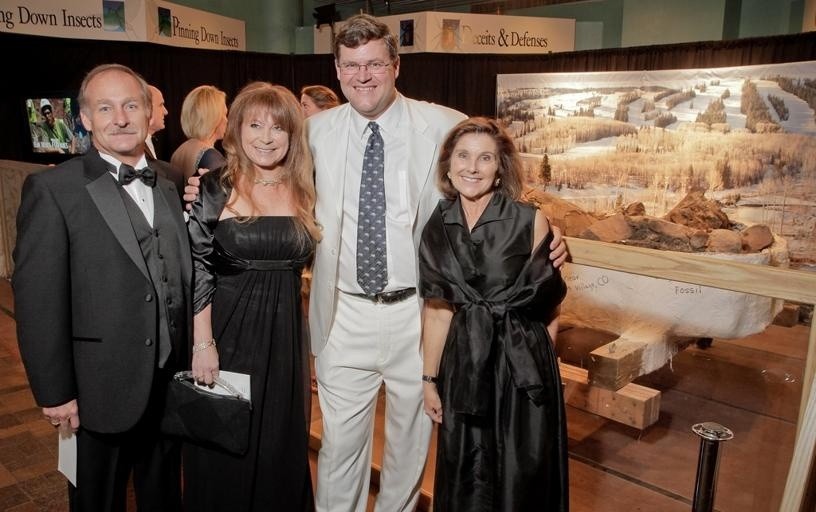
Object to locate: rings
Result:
[50,421,61,428]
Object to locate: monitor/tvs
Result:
[26,97,92,156]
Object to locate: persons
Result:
[171,86,229,182]
[144,85,183,188]
[184,14,471,511]
[421,118,570,512]
[11,65,193,512]
[298,86,340,118]
[39,104,78,153]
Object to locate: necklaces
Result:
[243,172,287,189]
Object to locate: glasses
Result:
[43,111,52,116]
[336,61,394,76]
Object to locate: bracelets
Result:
[190,336,216,357]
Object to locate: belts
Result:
[334,286,416,304]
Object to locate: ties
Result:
[356,121,388,301]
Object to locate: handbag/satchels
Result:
[150,371,252,472]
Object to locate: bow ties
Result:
[104,159,159,192]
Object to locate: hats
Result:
[26,99,32,109]
[40,99,53,113]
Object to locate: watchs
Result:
[422,375,440,386]
